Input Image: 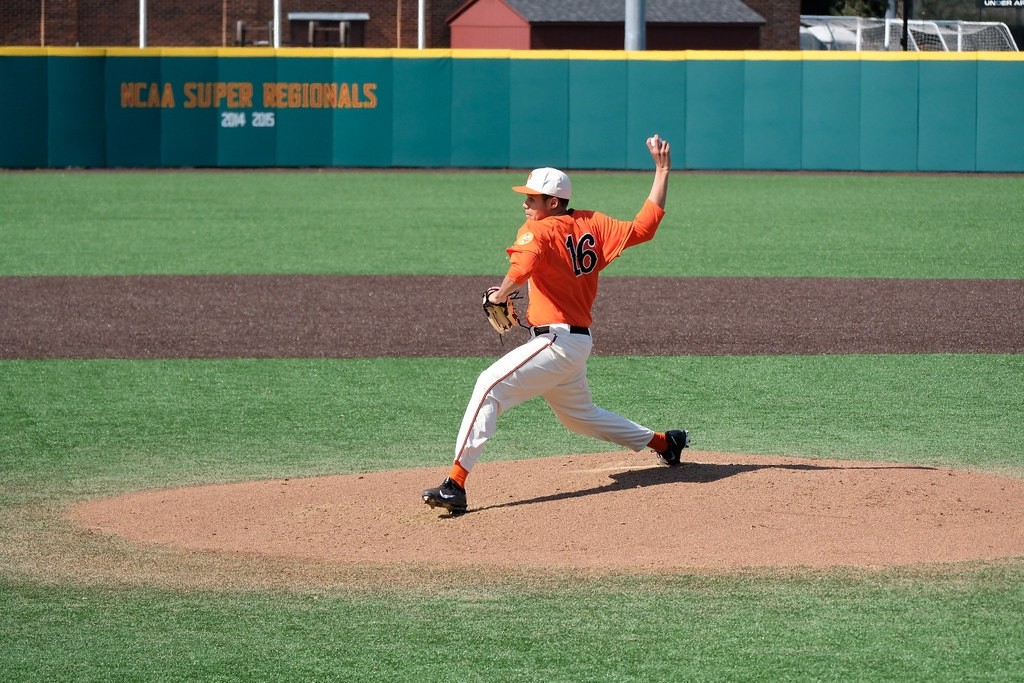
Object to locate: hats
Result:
[512,166,571,200]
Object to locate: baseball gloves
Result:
[481,286,521,334]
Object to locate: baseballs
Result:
[650,136,662,147]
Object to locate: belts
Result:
[534,326,589,335]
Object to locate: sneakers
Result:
[422,477,468,513]
[658,429,690,466]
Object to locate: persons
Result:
[423,133,690,515]
